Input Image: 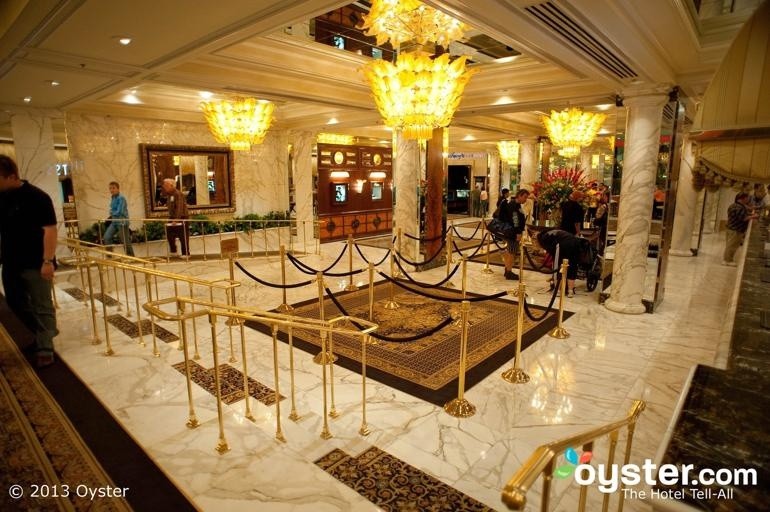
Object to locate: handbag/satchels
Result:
[487,218,516,242]
[493,199,506,218]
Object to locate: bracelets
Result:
[43,258,53,265]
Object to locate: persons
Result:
[0,154,59,367]
[488,182,612,298]
[162,178,193,261]
[721,182,770,267]
[103,181,135,259]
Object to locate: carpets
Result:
[0,284,204,512]
[242,274,580,408]
[342,218,613,279]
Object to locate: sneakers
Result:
[721,260,737,266]
[567,290,574,298]
[505,272,519,280]
[37,351,54,368]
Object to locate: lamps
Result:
[355,4,489,149]
[536,107,609,160]
[198,96,276,152]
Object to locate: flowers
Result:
[527,161,613,210]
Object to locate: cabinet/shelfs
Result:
[318,211,392,239]
[317,143,393,171]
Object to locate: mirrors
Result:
[139,142,237,219]
[603,109,676,302]
[419,127,447,271]
